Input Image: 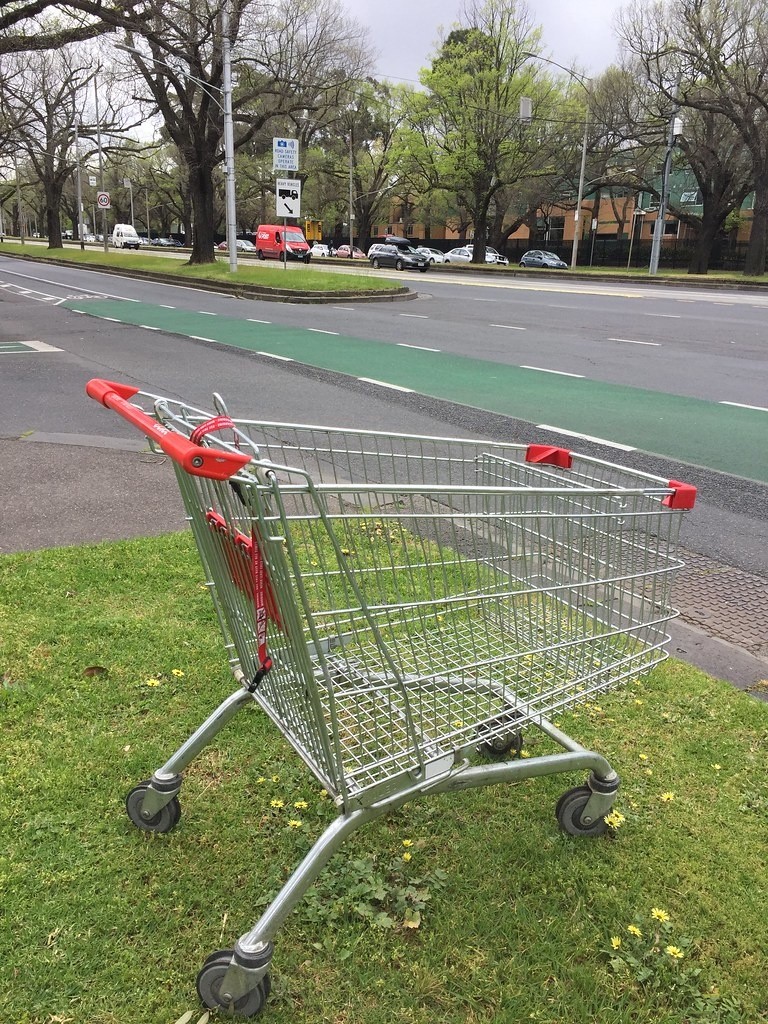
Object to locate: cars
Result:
[366,244,384,259]
[61,229,114,241]
[443,248,494,268]
[519,250,569,270]
[311,244,336,258]
[140,237,184,248]
[416,246,446,265]
[235,239,257,253]
[465,243,510,267]
[218,242,226,250]
[33,232,40,238]
[336,245,367,259]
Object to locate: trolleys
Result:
[84,377,698,1021]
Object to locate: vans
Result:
[113,223,141,250]
[256,224,311,263]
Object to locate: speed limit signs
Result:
[98,194,111,206]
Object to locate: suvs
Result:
[368,237,432,273]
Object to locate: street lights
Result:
[293,115,354,257]
[523,51,595,269]
[30,147,86,251]
[114,41,239,274]
